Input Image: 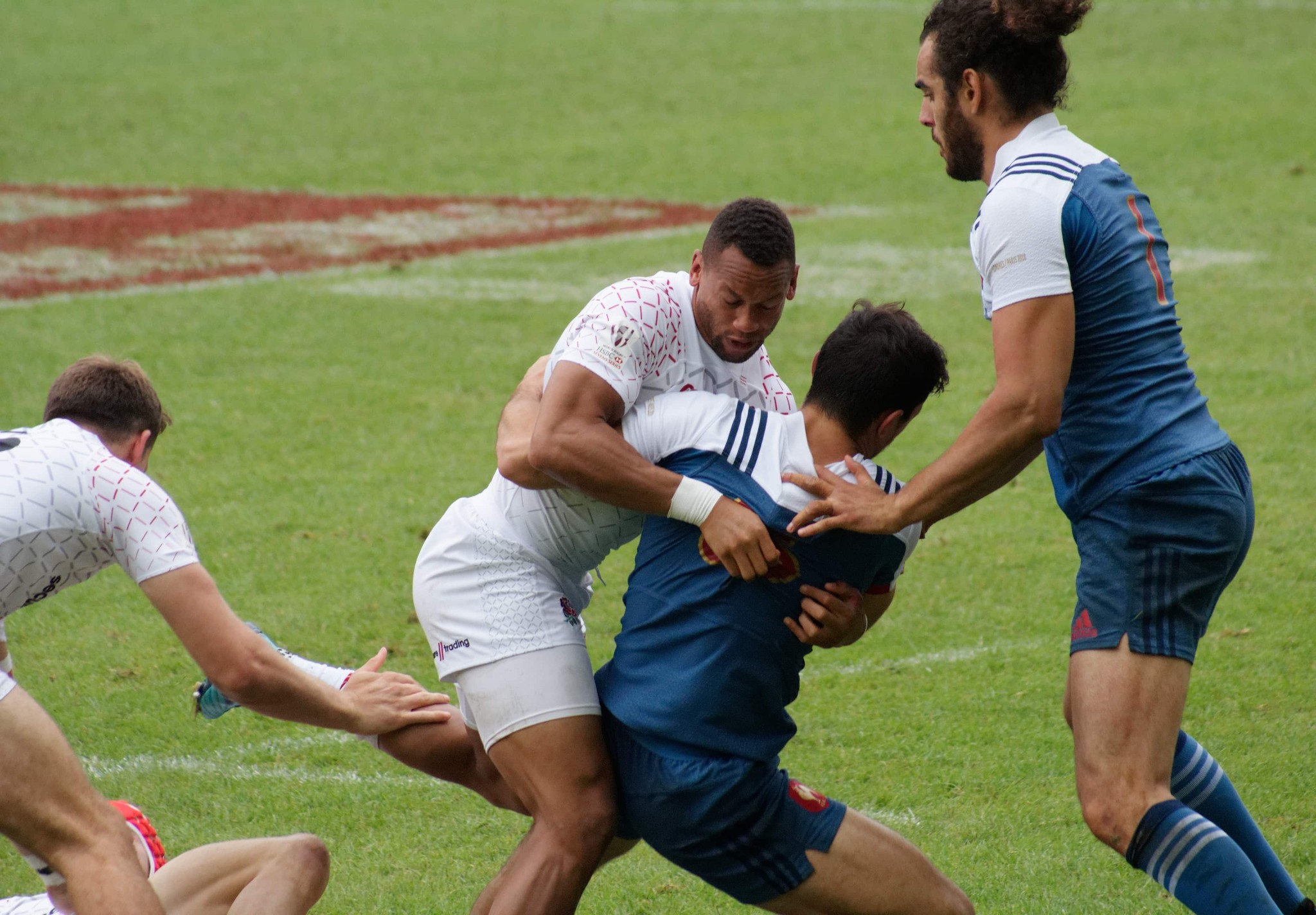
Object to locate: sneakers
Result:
[192,622,277,719]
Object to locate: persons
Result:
[0,357,451,915]
[0,802,329,915]
[194,197,897,915]
[780,0,1316,915]
[471,301,975,915]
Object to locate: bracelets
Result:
[863,613,867,636]
[667,475,723,526]
